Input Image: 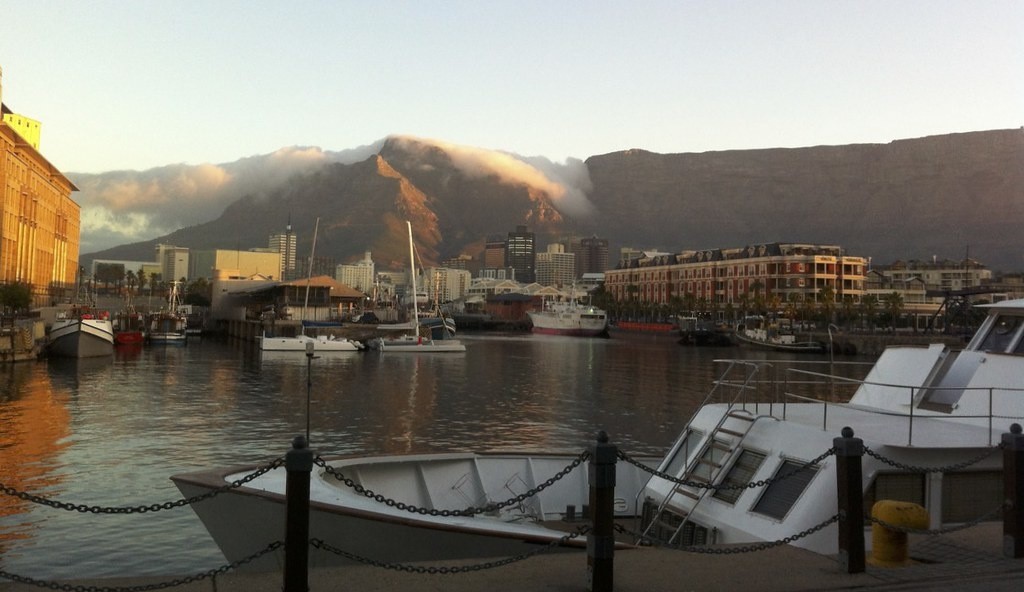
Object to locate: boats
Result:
[42,274,114,358]
[437,291,535,332]
[168,298,1023,592]
[147,280,188,340]
[112,291,147,345]
[525,280,609,336]
[735,328,824,354]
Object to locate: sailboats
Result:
[254,216,366,350]
[364,220,467,352]
